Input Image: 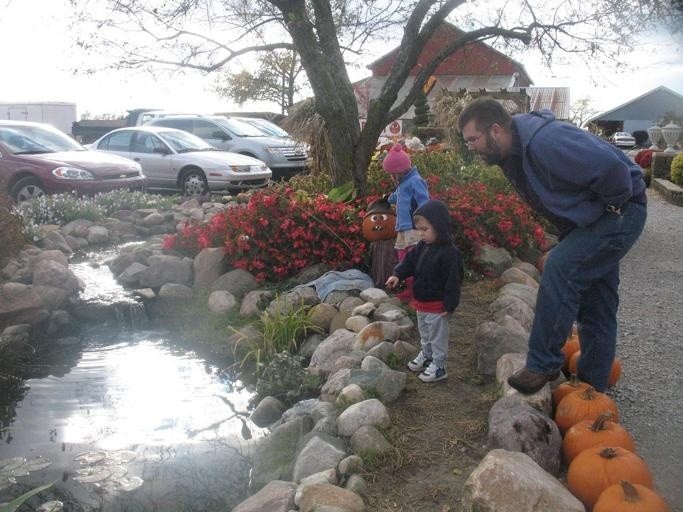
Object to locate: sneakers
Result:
[507,358,562,396]
[406,350,432,371]
[418,361,448,384]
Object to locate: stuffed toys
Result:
[360,198,399,289]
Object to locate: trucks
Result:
[72,107,165,145]
[0,101,78,141]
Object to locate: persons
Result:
[456,96,649,394]
[383,143,429,296]
[382,201,463,383]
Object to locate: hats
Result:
[381,142,414,174]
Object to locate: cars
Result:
[0,117,149,209]
[610,131,637,149]
[83,111,314,197]
